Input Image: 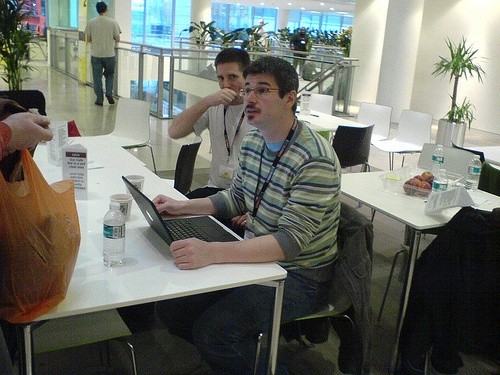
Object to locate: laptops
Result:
[121,176,240,247]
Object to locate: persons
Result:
[290,28,310,76]
[156,57,340,375]
[84,1,122,105]
[168,48,258,239]
[0,111,53,159]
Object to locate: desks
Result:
[297,107,369,130]
[340,164,500,375]
[22,134,289,374]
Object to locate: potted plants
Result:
[430,35,486,147]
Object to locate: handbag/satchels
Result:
[0,149,81,325]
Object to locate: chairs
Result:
[371,108,433,172]
[34,309,137,374]
[102,98,157,174]
[356,101,392,140]
[161,135,203,192]
[251,279,355,375]
[418,140,477,239]
[0,90,47,118]
[330,123,377,205]
[308,93,334,114]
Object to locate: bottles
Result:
[103,202,126,268]
[464,155,483,197]
[432,169,448,192]
[430,144,446,177]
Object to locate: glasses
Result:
[238,86,283,96]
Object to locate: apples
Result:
[403,171,434,195]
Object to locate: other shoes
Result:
[107,96,115,104]
[95,102,103,106]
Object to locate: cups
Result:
[110,194,133,221]
[127,175,145,193]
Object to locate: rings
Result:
[244,220,246,223]
